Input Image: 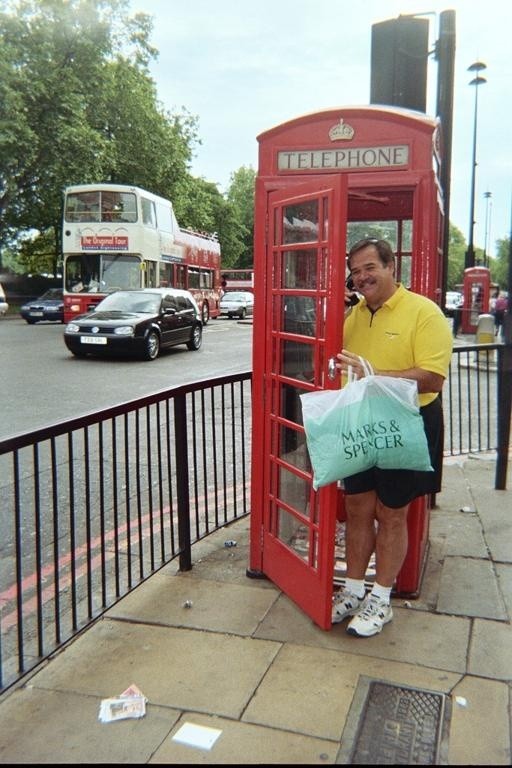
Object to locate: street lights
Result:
[465,60,493,268]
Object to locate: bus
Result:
[61,184,222,324]
[220,269,255,293]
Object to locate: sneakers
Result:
[331,588,393,637]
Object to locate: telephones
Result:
[345,272,360,307]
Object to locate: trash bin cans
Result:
[475,313,495,363]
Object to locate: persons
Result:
[493,294,509,336]
[78,207,97,221]
[310,235,456,640]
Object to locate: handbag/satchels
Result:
[299,355,435,492]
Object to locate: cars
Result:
[64,289,204,360]
[446,291,462,315]
[21,288,63,324]
[218,292,253,319]
